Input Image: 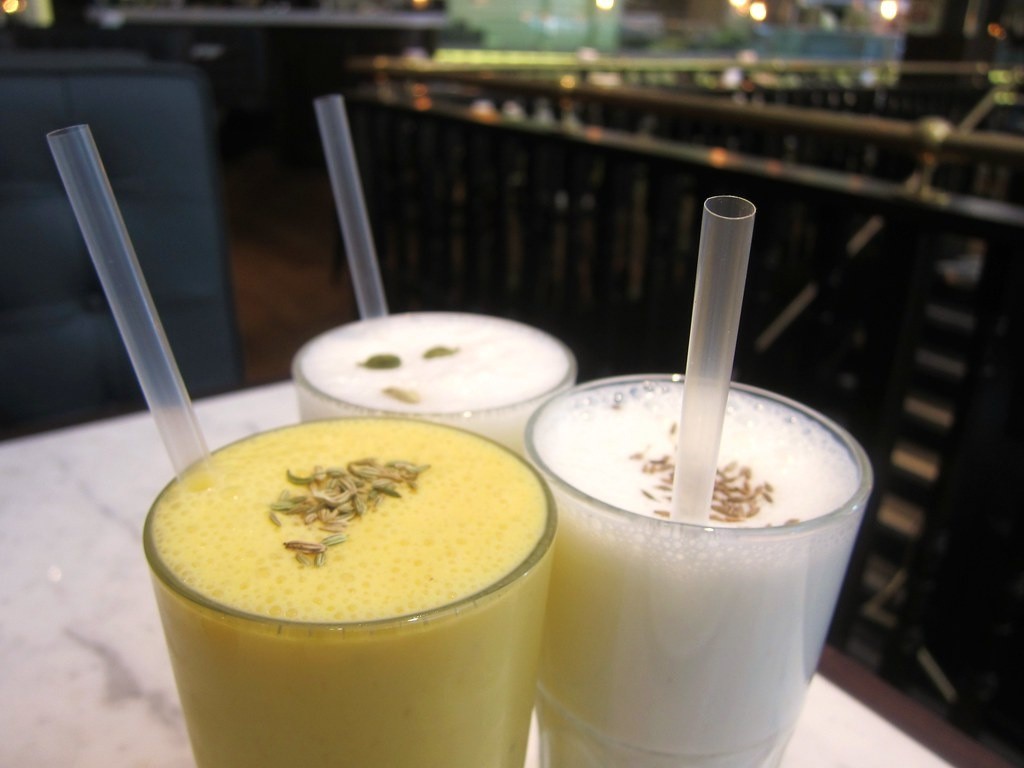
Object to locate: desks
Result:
[0,383,1024,768]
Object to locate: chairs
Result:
[1,51,250,444]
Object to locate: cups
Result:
[293,314,578,454]
[524,371,873,767]
[143,414,556,767]
